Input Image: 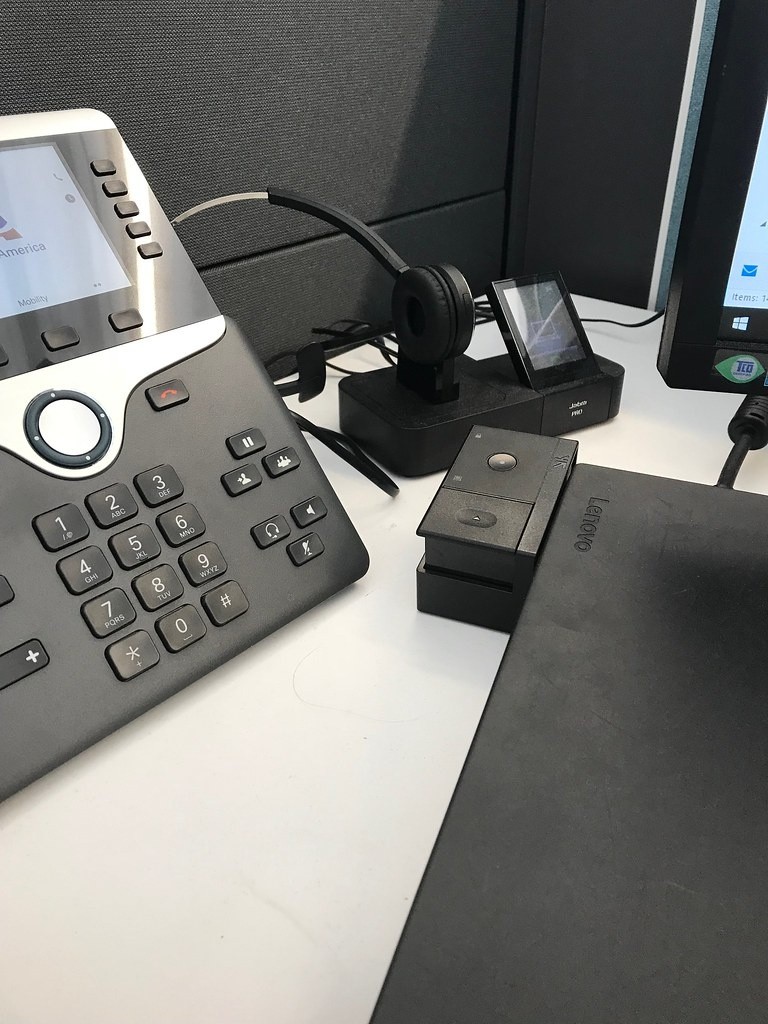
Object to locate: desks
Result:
[0,292,768,1024]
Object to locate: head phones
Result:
[165,188,475,407]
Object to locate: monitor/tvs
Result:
[653,0,768,392]
